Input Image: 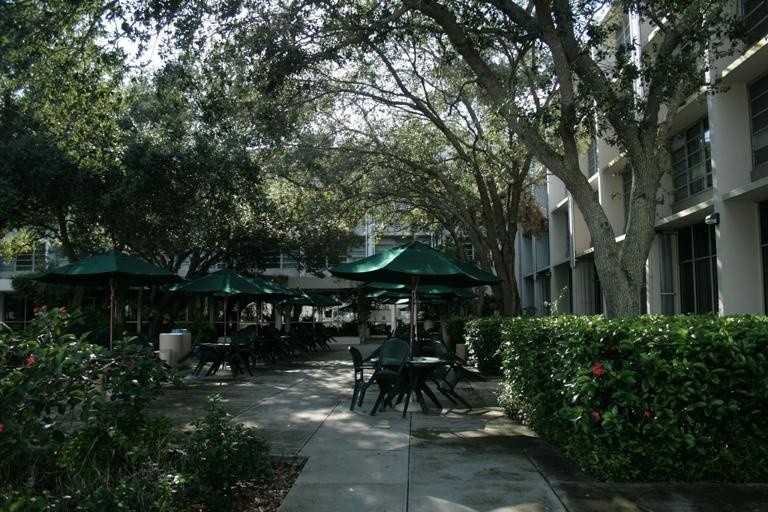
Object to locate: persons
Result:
[149,312,178,349]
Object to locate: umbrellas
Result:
[29,249,192,351]
[328,240,507,356]
[176,267,342,342]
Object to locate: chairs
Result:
[40,316,337,377]
[344,324,474,419]
